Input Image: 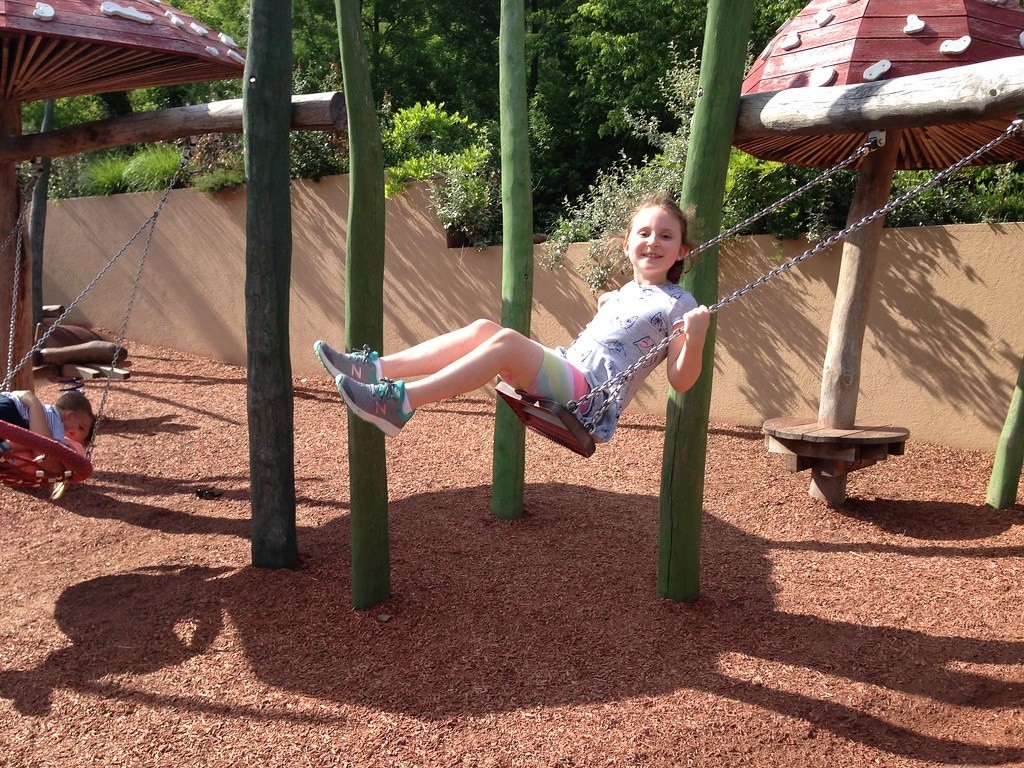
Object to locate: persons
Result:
[314,194,710,443]
[0,390,95,470]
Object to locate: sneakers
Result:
[314,340,384,385]
[336,374,415,438]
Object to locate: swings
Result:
[494,114,1023,459]
[1,144,193,488]
